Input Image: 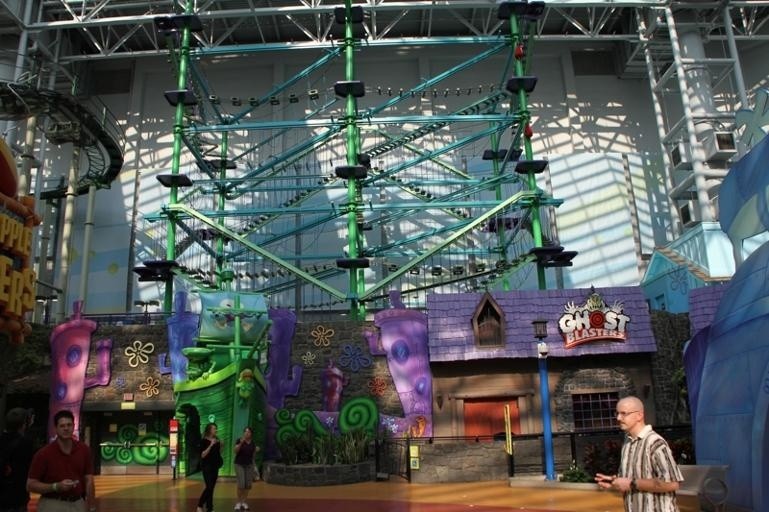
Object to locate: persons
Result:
[194,422,224,512]
[0,407,40,512]
[25,409,98,512]
[233,426,260,510]
[595,394,686,512]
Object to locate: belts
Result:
[53,495,81,502]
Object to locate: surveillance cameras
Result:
[539,343,549,357]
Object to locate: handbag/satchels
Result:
[253,461,260,480]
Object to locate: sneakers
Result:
[196,506,203,512]
[234,502,241,510]
[242,503,249,510]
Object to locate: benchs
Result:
[675,466,731,509]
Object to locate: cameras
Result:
[67,480,80,489]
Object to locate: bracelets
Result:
[51,482,60,494]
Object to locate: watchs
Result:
[630,476,640,495]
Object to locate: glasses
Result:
[613,412,638,417]
